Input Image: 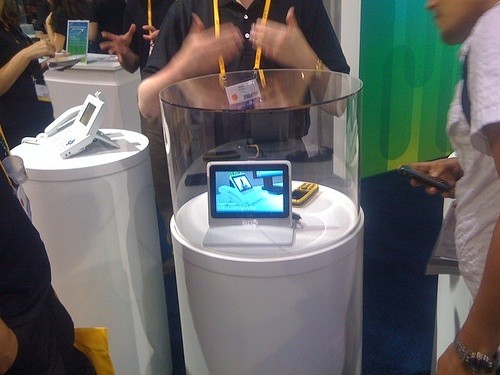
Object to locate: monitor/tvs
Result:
[206,161,293,229]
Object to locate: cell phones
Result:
[399,165,451,191]
[291,182,319,206]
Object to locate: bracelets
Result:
[315,58,324,79]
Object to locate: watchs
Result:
[453,333,494,370]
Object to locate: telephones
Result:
[40,92,121,159]
[218,173,262,206]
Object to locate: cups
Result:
[2,156,28,184]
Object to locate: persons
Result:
[0,0,55,152]
[138,0,351,162]
[32,0,175,262]
[0,180,98,375]
[406,0,500,375]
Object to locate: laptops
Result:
[230,173,266,203]
